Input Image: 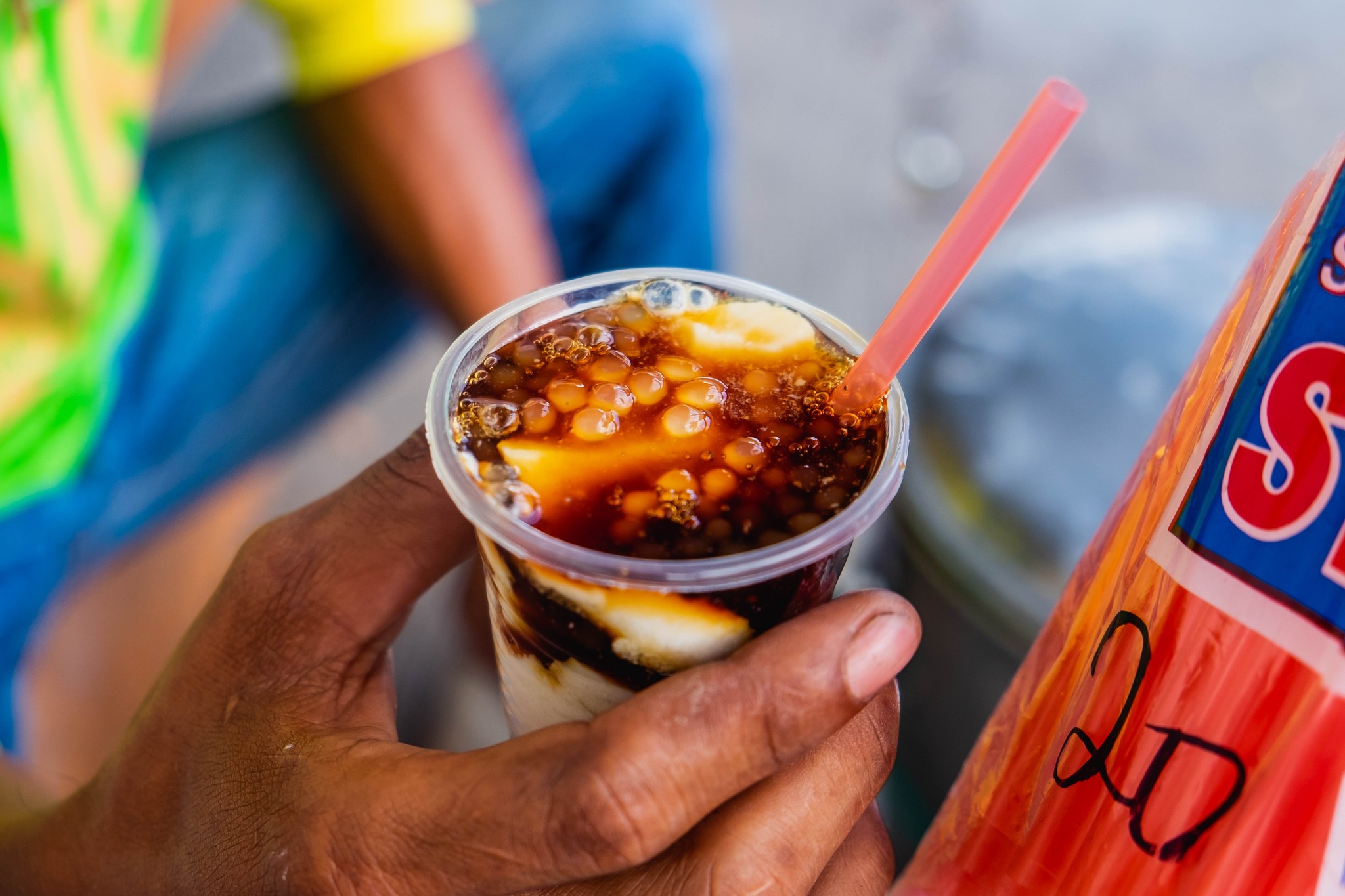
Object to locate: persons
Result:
[0,2,924,896]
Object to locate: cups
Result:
[424,267,909,744]
